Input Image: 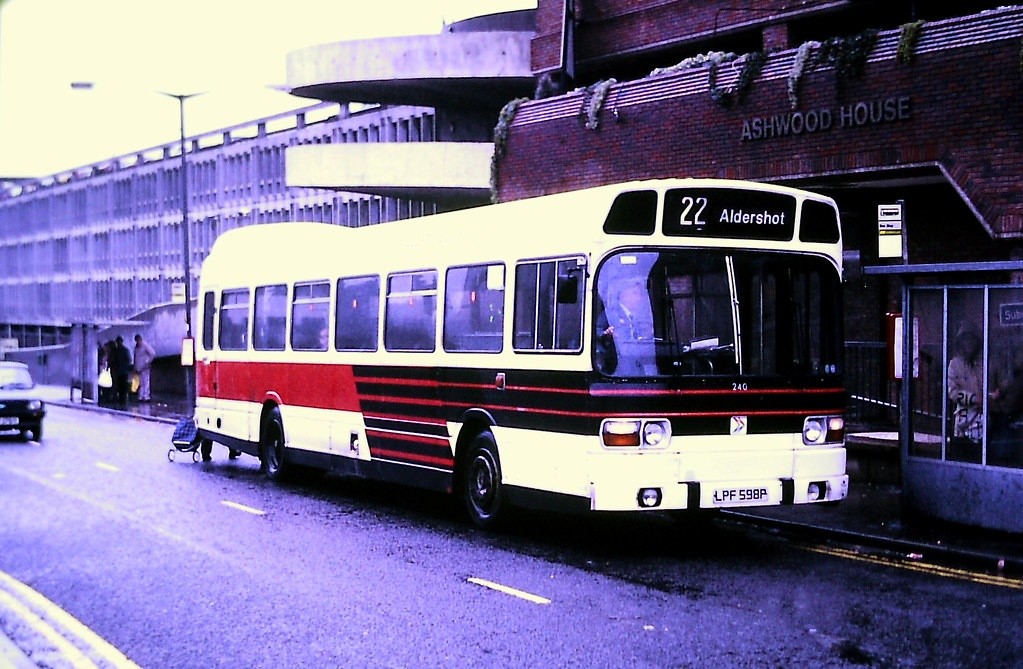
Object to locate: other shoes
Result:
[203,455,212,461]
[229,450,241,459]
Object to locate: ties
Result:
[630,315,637,339]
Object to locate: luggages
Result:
[167,414,200,462]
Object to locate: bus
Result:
[192,176,851,516]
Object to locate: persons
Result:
[132,334,155,401]
[945,328,1008,465]
[318,327,328,349]
[97,335,133,411]
[201,439,242,462]
[596,281,643,338]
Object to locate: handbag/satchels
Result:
[98,362,113,388]
[131,374,140,393]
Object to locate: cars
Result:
[0,360,46,442]
